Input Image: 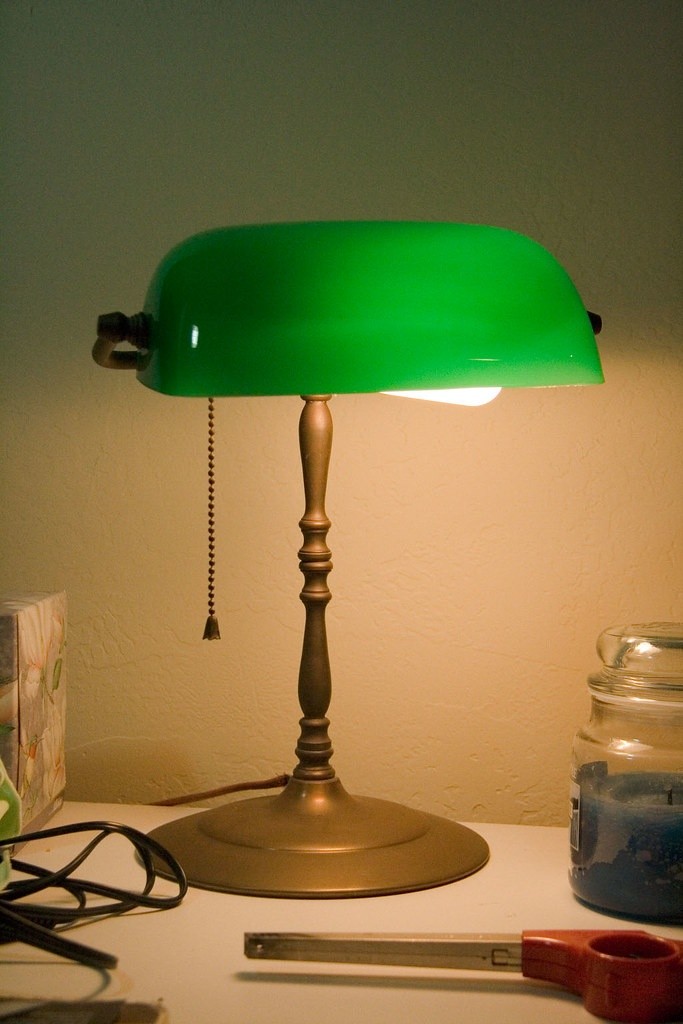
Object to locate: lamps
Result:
[93,218,606,897]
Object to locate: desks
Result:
[0,801,683,1024]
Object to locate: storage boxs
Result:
[1,590,70,859]
